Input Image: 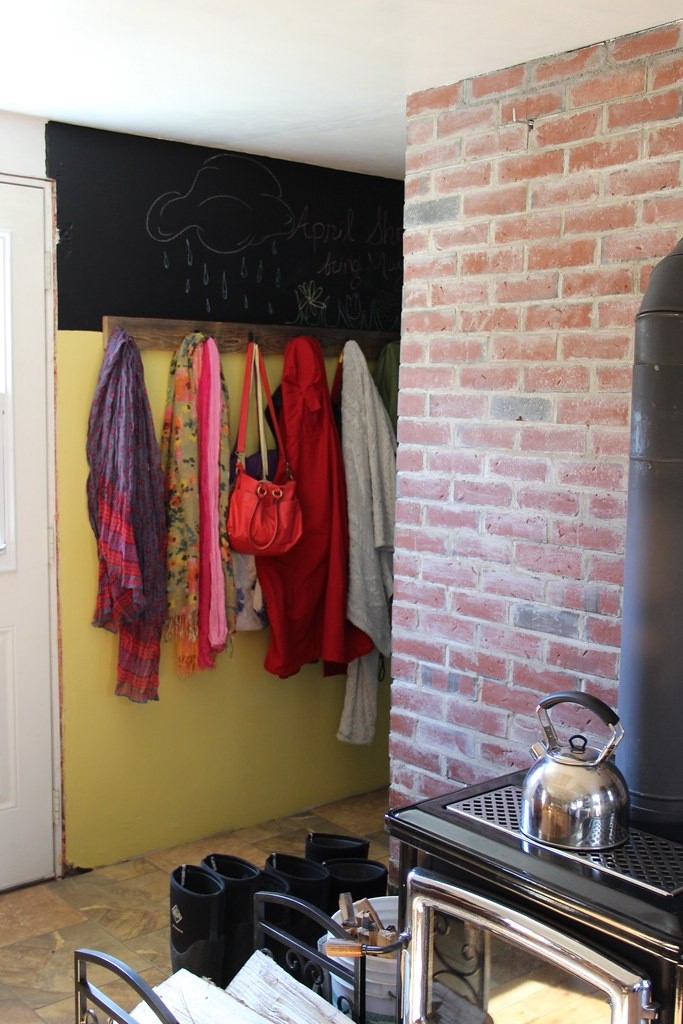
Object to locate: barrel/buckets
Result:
[318,896,397,1024]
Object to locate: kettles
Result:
[518,692,630,851]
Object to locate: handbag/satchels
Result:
[228,447,280,484]
[226,471,305,556]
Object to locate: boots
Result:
[169,831,391,987]
